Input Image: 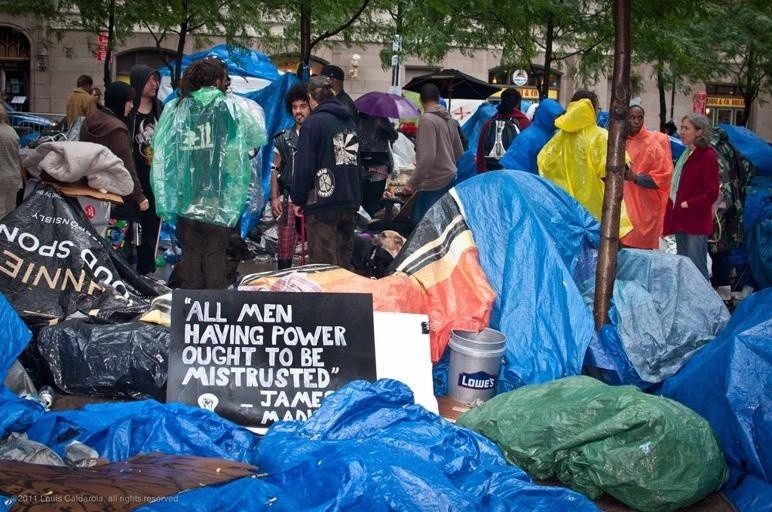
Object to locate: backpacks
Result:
[479,112,521,170]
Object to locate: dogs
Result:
[370,229,408,259]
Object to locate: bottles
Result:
[130,216,146,248]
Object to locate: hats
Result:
[308,63,345,81]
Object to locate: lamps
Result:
[348,51,361,80]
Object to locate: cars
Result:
[2,101,54,130]
